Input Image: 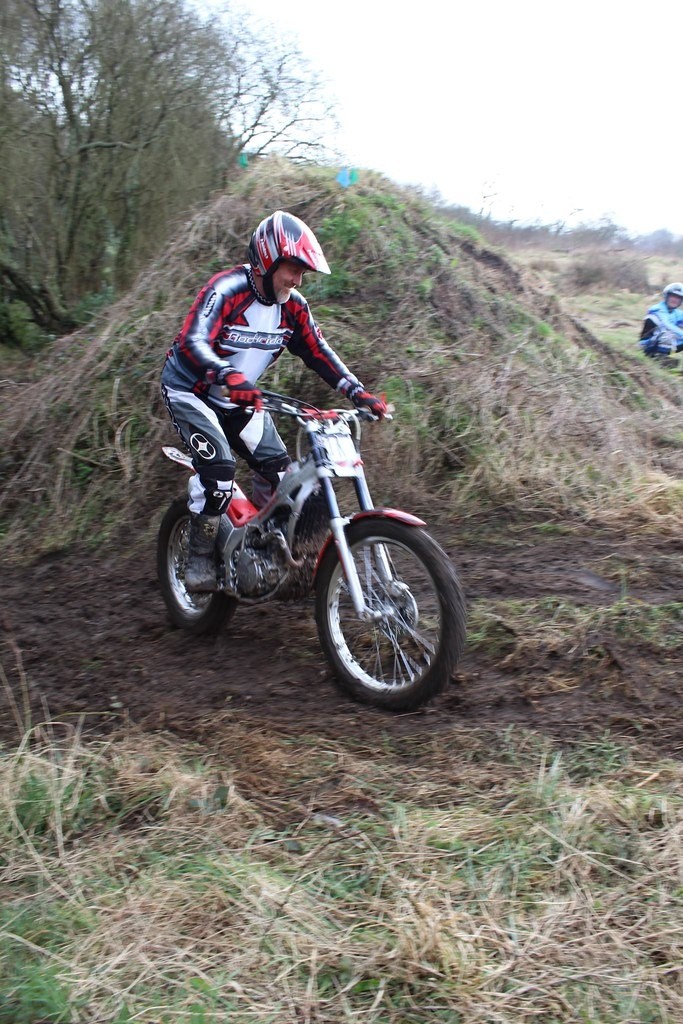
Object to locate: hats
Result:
[663,282,683,301]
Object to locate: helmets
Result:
[248,211,332,278]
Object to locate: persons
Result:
[160,210,397,605]
[639,280,683,361]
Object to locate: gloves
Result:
[225,372,262,412]
[354,391,387,421]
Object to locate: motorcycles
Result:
[156,384,466,712]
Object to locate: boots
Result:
[185,513,220,593]
